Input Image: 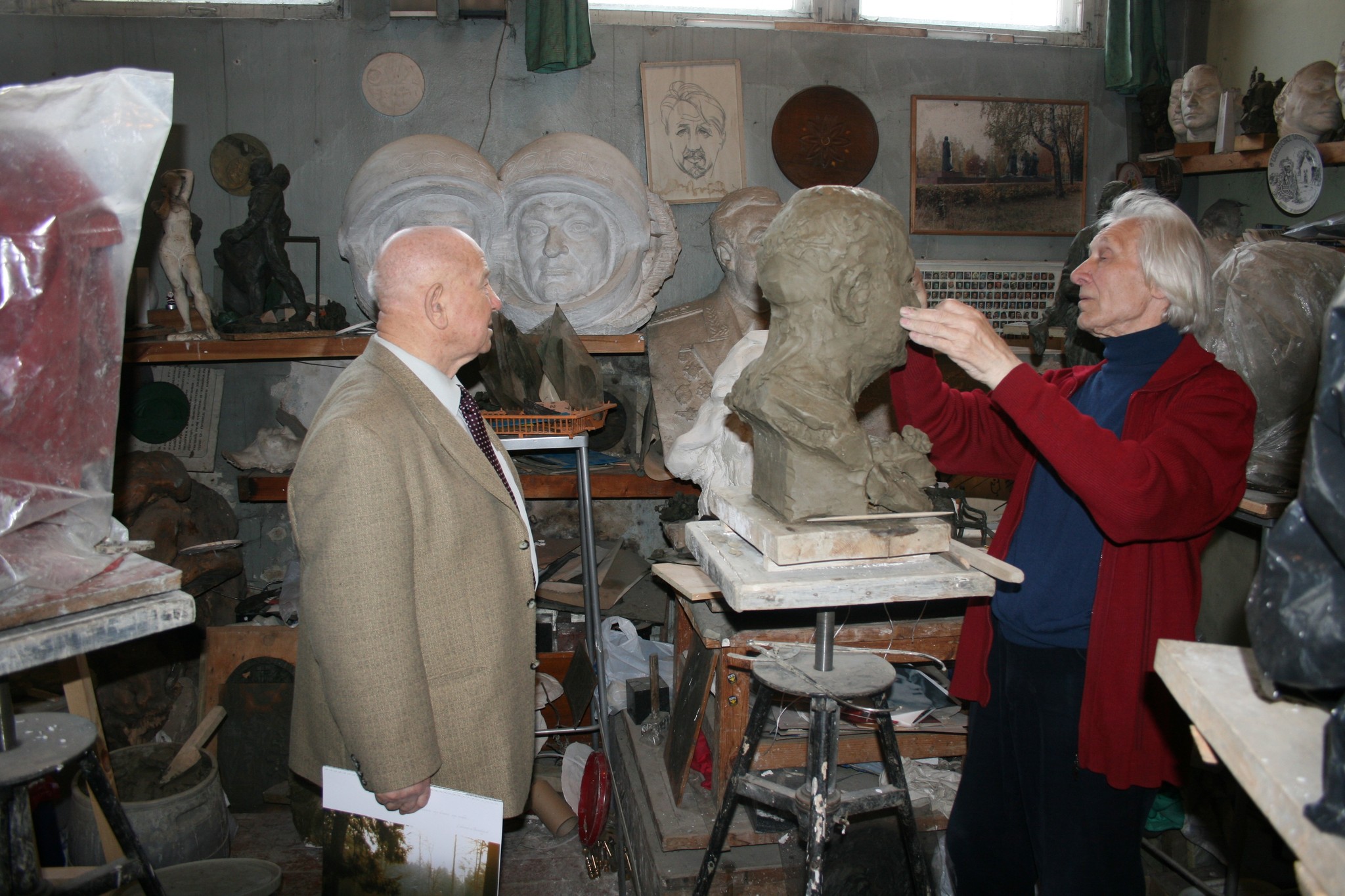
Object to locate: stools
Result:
[497,433,613,753]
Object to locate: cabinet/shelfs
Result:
[116,324,697,500]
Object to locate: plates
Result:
[1155,157,1183,205]
[1116,161,1143,191]
[1267,133,1323,214]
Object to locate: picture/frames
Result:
[638,58,749,205]
[910,95,1088,236]
[282,236,322,331]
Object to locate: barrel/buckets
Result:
[67,741,231,870]
[100,857,283,896]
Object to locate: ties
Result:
[457,384,519,519]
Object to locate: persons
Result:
[1005,149,1019,176]
[1196,198,1244,267]
[1054,180,1130,371]
[1273,40,1345,144]
[899,188,1258,896]
[647,186,940,521]
[918,270,1054,339]
[1021,149,1038,176]
[213,156,310,329]
[285,224,542,896]
[1167,64,1224,143]
[338,134,680,334]
[150,168,221,342]
[941,136,954,171]
